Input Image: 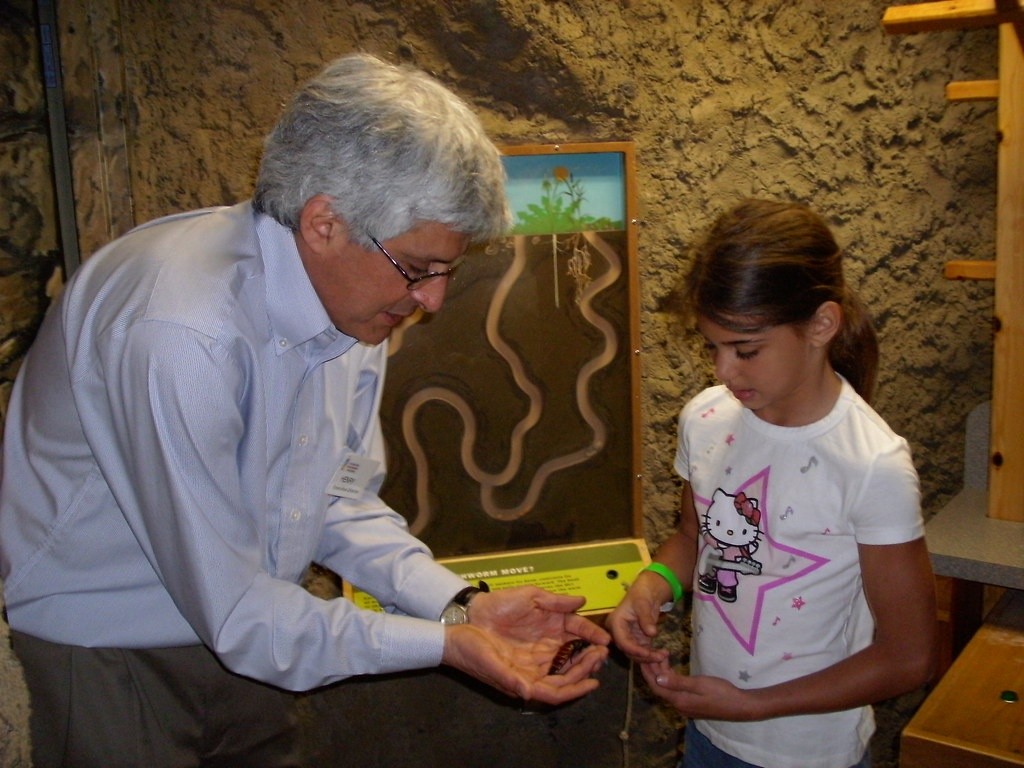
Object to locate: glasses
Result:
[364,229,460,292]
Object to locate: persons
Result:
[0,53,611,768]
[607,201,943,768]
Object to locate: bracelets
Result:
[639,561,681,611]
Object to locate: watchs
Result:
[441,580,489,625]
